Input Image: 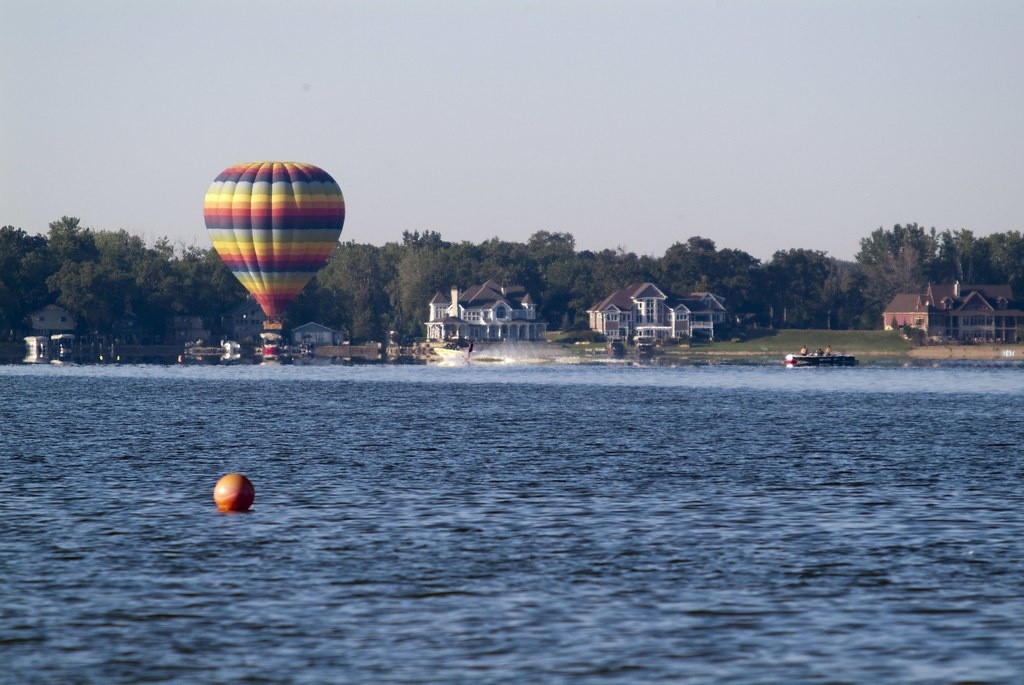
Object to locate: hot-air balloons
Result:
[203,160,346,362]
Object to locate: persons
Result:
[800,345,831,356]
[467,341,473,358]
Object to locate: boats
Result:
[785,353,856,367]
